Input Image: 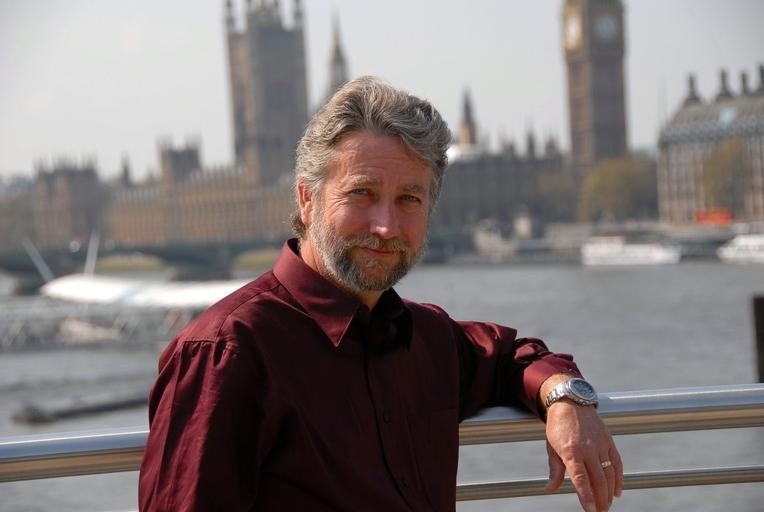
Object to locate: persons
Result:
[140,73,624,511]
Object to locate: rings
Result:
[599,458,613,469]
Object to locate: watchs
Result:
[540,377,601,408]
